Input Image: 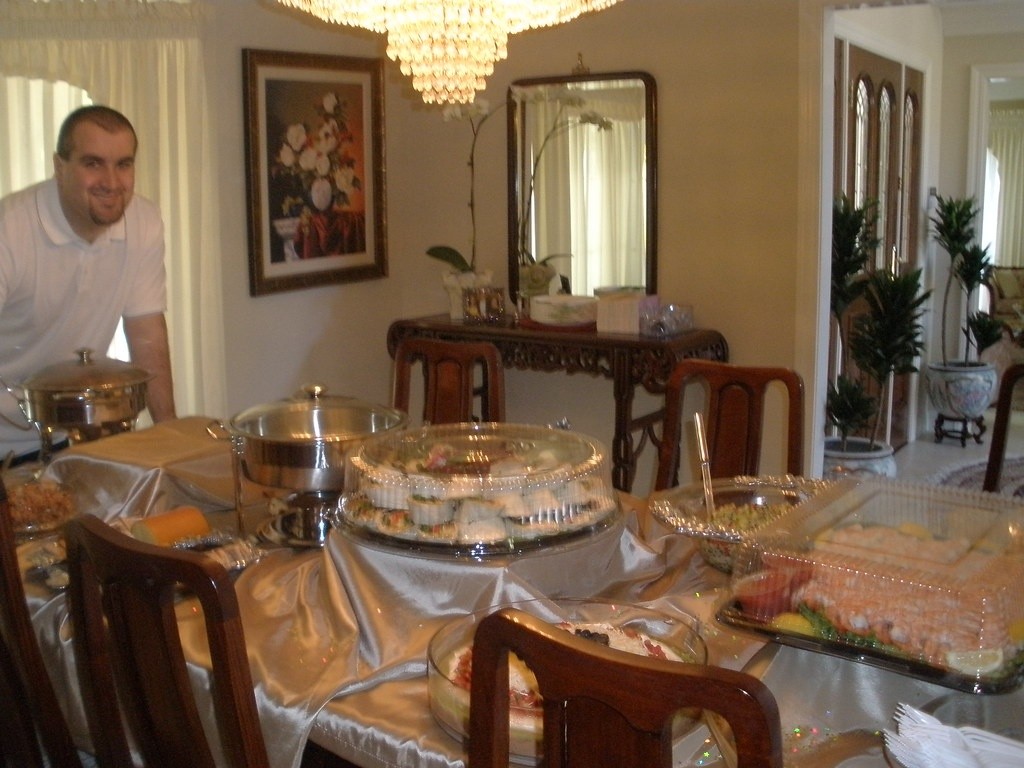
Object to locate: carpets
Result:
[926,457,1024,552]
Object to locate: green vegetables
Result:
[797,601,913,660]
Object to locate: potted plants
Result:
[924,194,1004,418]
[823,198,934,483]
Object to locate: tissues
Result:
[529,273,597,327]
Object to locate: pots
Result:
[0,348,156,430]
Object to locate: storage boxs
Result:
[597,296,643,336]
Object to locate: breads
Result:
[129,505,210,545]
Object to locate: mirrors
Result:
[507,71,658,306]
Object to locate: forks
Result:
[884,701,1024,768]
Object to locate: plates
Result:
[12,510,74,544]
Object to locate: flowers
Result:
[427,80,542,272]
[519,92,615,264]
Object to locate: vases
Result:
[439,270,494,321]
[517,265,563,301]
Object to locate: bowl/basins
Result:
[428,598,709,765]
[46,558,259,608]
[647,476,828,573]
[639,295,693,336]
[529,295,599,326]
[208,382,411,494]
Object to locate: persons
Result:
[0,105,178,465]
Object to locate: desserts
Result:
[346,440,610,543]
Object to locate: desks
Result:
[389,310,730,493]
[0,414,1024,768]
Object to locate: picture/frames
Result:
[463,287,506,326]
[242,48,389,298]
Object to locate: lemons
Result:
[899,524,936,543]
[770,611,815,635]
[944,647,1004,677]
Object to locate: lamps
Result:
[277,0,624,105]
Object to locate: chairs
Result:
[1,337,803,768]
